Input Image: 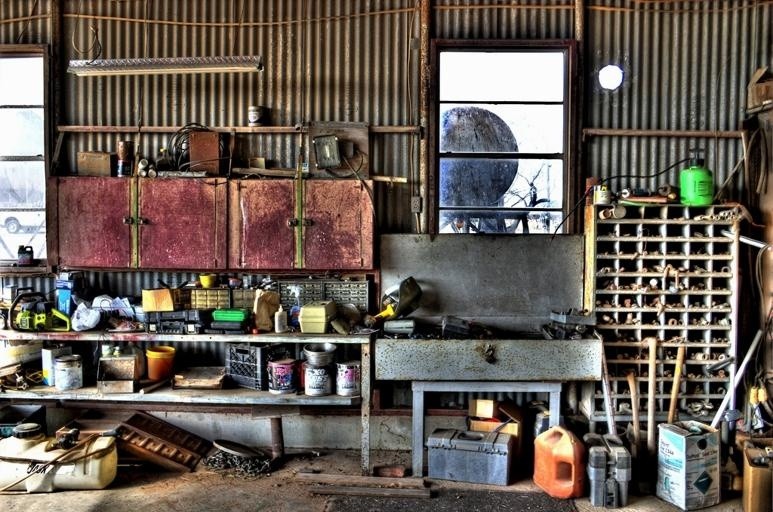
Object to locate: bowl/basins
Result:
[304,343,337,367]
[200,275,216,288]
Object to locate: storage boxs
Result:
[427,395,524,486]
[656,419,773,512]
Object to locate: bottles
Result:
[53,354,84,392]
[275,305,286,332]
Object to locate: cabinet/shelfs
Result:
[0,330,377,477]
[226,179,373,270]
[411,380,562,478]
[582,202,740,437]
[46,177,226,273]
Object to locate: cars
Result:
[0,211,46,233]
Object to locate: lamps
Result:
[67,0,265,77]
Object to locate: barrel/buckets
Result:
[301,361,331,396]
[335,359,361,396]
[533,425,586,499]
[265,359,296,394]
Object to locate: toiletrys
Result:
[275,303,288,333]
[679,148,715,207]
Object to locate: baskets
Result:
[224,342,296,390]
